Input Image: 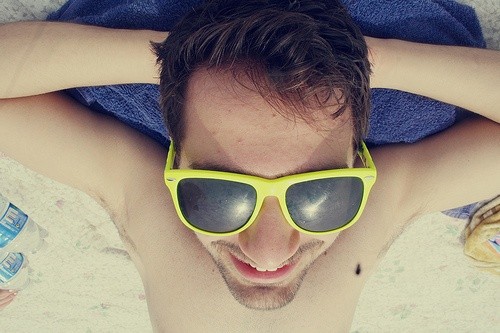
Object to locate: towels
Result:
[44,1,488,221]
[463,193,500,279]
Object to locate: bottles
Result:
[0,194,45,294]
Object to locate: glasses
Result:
[165,135,376,235]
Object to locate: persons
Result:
[0,0,500,333]
